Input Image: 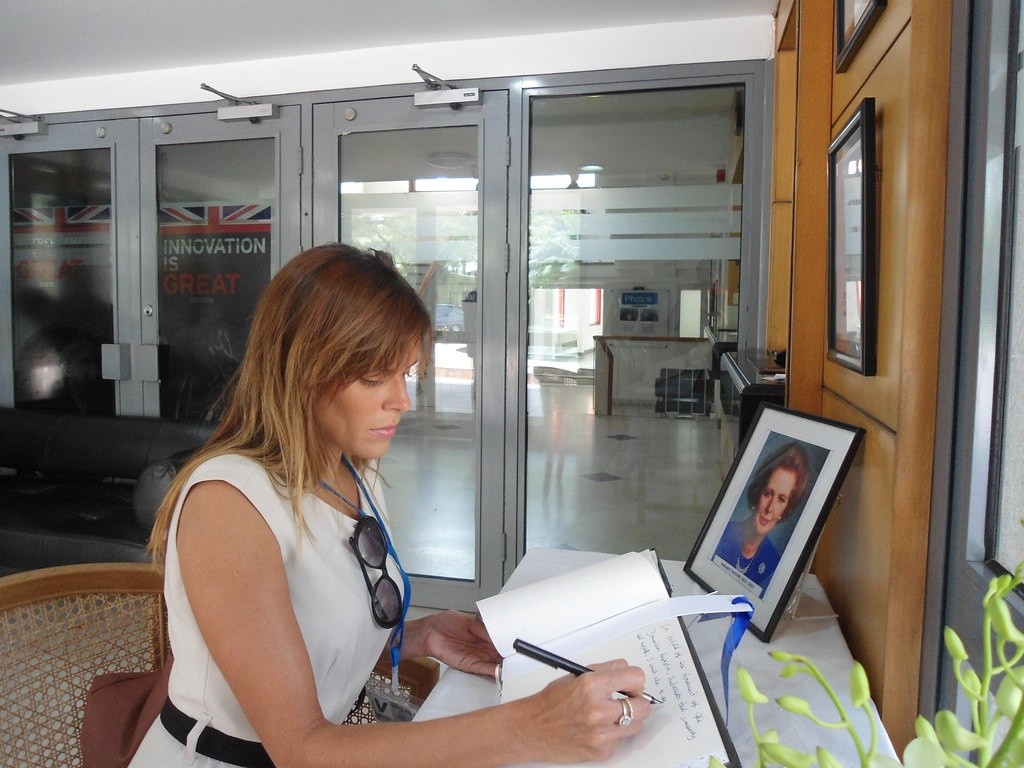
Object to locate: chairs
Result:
[0,562,440,767]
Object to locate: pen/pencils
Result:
[513,639,662,705]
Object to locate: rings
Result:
[617,699,634,728]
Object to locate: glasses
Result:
[349,516,401,628]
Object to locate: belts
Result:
[159,696,275,768]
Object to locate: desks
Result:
[410,545,906,766]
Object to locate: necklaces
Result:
[735,522,761,576]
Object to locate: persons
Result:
[711,441,809,601]
[124,242,650,766]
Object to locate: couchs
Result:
[0,409,220,579]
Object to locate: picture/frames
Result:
[826,96,876,377]
[833,0,888,75]
[681,400,868,643]
